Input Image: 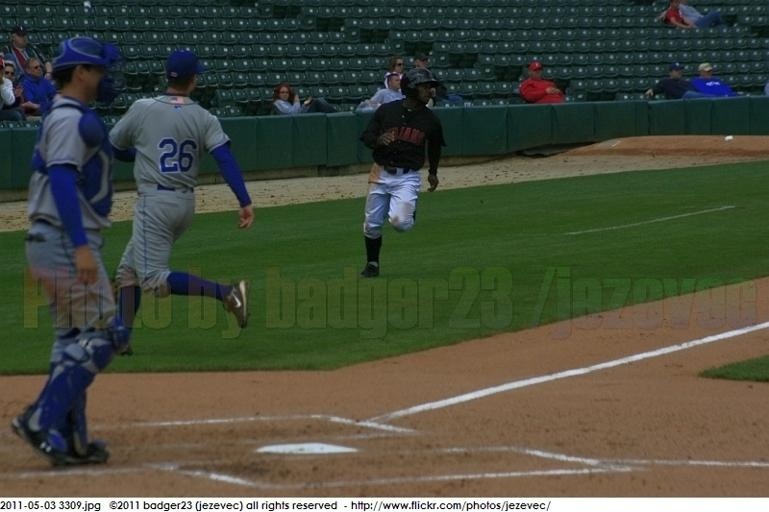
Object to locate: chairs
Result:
[1,1,768,128]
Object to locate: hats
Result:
[414,53,427,60]
[12,25,27,36]
[669,63,683,71]
[698,63,711,72]
[528,61,541,70]
[166,50,203,81]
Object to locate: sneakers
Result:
[11,408,107,466]
[360,263,379,277]
[224,280,248,328]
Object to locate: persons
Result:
[273,84,336,114]
[657,0,726,28]
[519,61,565,103]
[109,49,254,356]
[689,61,739,96]
[11,34,130,464]
[645,61,708,99]
[665,0,695,29]
[0,32,57,122]
[360,67,442,277]
[356,55,461,112]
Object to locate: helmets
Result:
[400,68,438,95]
[52,36,126,105]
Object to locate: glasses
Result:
[5,71,14,75]
